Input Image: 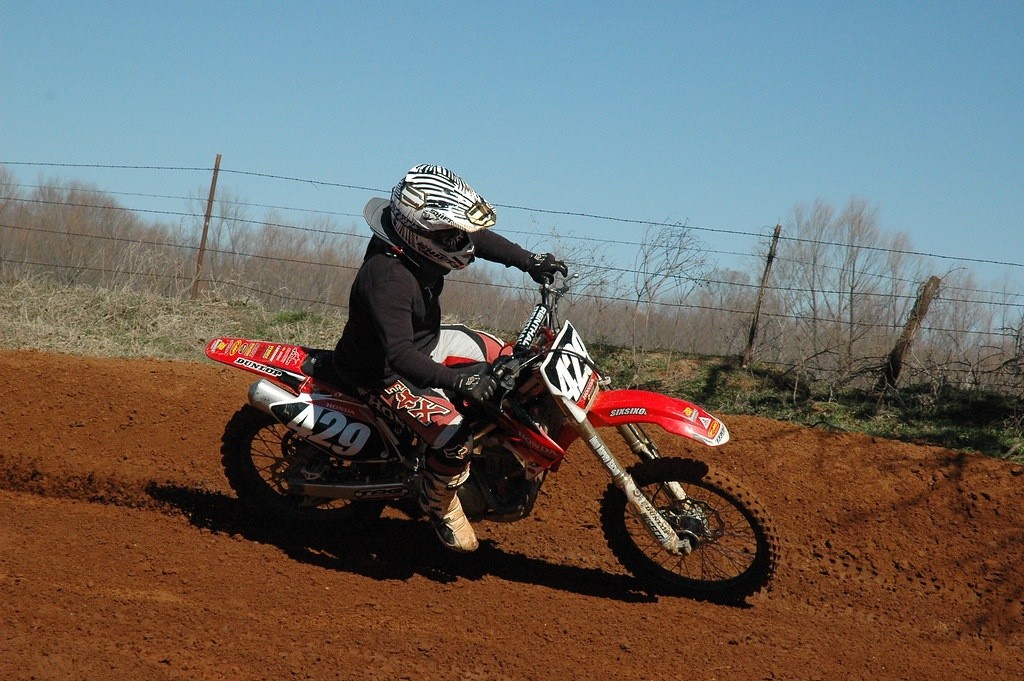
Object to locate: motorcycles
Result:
[204,271,781,607]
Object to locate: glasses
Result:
[429,227,470,253]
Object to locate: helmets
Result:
[390,164,496,269]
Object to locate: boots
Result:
[418,446,480,553]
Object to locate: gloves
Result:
[454,373,497,408]
[524,253,568,285]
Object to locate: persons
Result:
[329,164,568,554]
